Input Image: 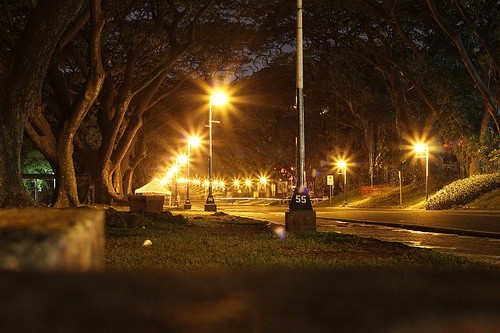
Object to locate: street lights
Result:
[158,150,184,206]
[413,142,431,207]
[200,88,225,213]
[230,177,241,190]
[336,160,348,205]
[243,177,258,190]
[258,174,281,202]
[185,134,200,211]
[201,176,227,191]
